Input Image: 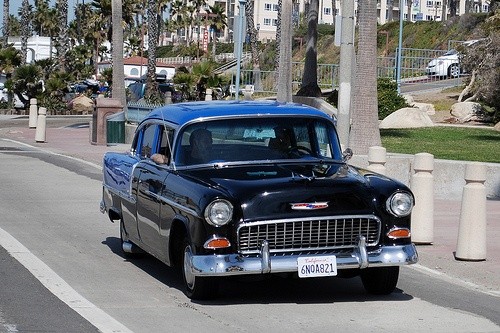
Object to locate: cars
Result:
[98,101,419,299]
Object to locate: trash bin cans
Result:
[106,111,126,146]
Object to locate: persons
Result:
[150,129,213,167]
[265,129,302,159]
[97,82,108,94]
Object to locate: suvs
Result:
[128,74,178,99]
[426,41,486,78]
